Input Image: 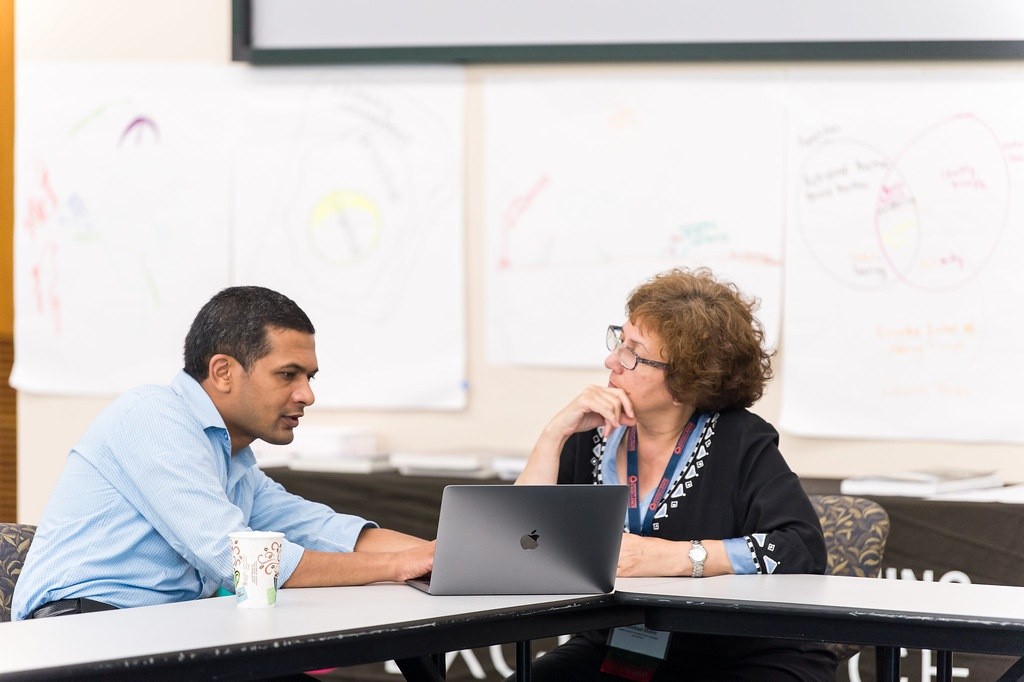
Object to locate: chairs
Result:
[804,495,891,664]
[0,523,38,623]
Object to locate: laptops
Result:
[405,484,630,594]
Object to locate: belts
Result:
[24,598,118,622]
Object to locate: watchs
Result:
[688,539,708,578]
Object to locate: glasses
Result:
[606,325,671,370]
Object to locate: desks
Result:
[0,573,1024,682]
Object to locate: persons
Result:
[8,283,437,622]
[507,267,839,682]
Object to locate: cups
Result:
[228,532,284,607]
